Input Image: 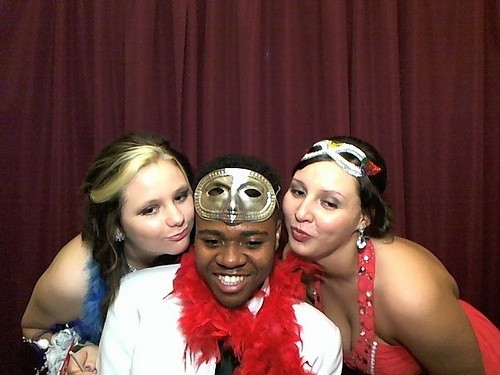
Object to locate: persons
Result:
[96,154,343,375]
[282,136,500,375]
[21,133,194,375]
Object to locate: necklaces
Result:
[127,260,136,272]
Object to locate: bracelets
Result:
[73,340,95,353]
[32,329,49,340]
